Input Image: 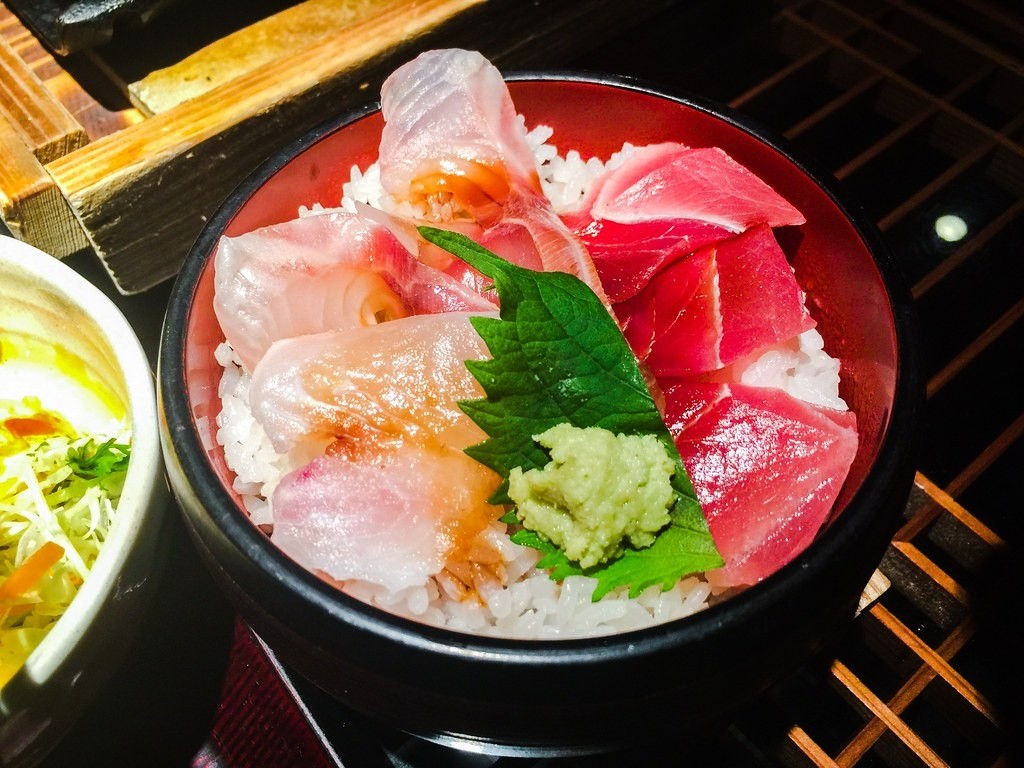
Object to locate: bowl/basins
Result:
[1,233,187,766]
[152,73,925,758]
